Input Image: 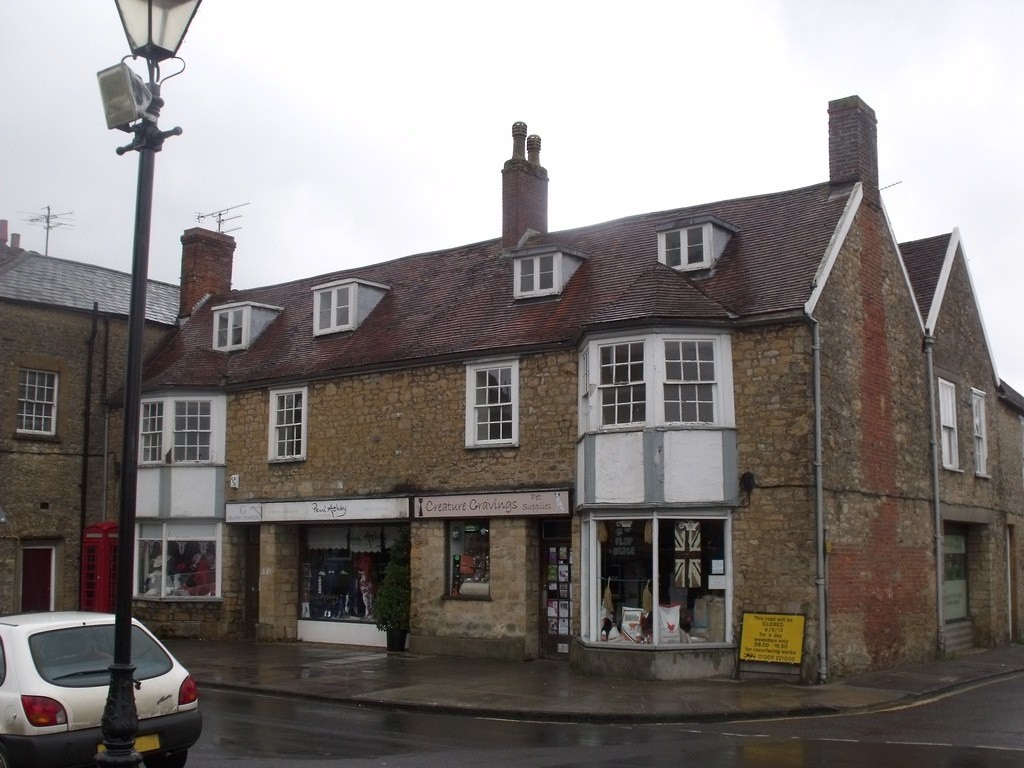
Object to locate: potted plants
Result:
[368,528,412,654]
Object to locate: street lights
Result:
[85,0,207,768]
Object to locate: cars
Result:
[1,607,204,768]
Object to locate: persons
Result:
[648,546,675,621]
